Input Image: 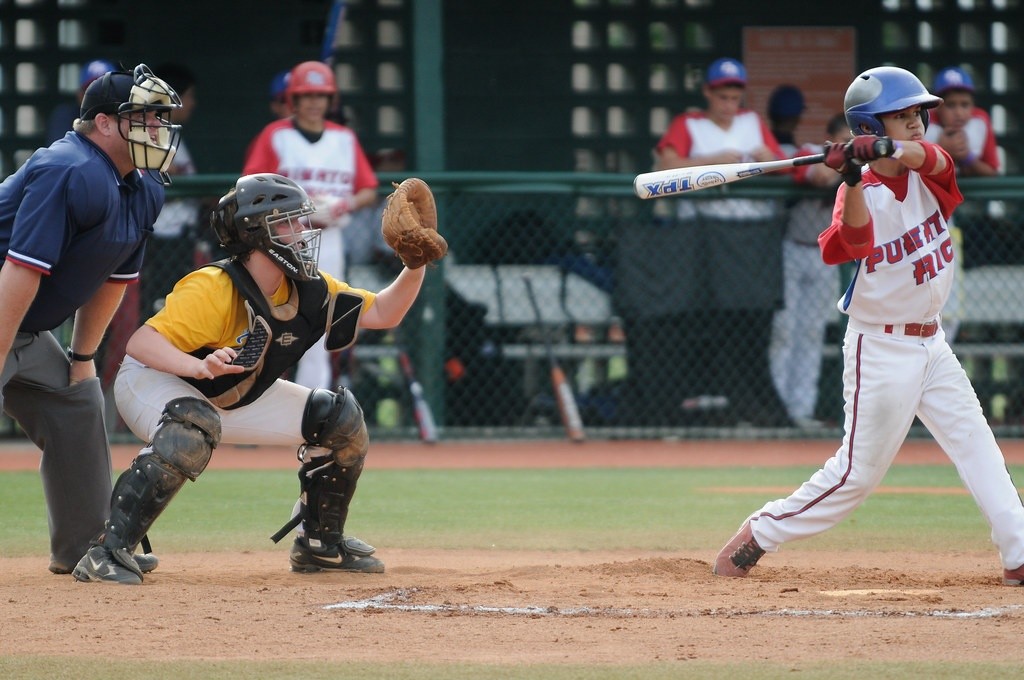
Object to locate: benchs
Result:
[346,262,631,357]
[821,261,1024,357]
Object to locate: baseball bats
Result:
[522,271,587,442]
[631,138,890,201]
[392,326,438,444]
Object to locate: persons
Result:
[0,61,183,575]
[73,172,426,589]
[709,66,1024,586]
[650,59,1008,426]
[147,60,410,426]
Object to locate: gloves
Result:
[822,140,861,186]
[842,134,899,164]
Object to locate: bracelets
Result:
[911,139,936,174]
[960,150,976,167]
[67,345,96,364]
[892,140,902,159]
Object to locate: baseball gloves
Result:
[379,177,451,272]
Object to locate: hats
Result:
[269,72,289,96]
[707,57,746,87]
[80,74,174,121]
[769,87,807,117]
[933,67,974,96]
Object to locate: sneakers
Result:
[1003,562,1024,585]
[289,534,385,573]
[73,546,141,586]
[48,546,159,573]
[713,516,766,578]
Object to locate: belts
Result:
[300,221,328,230]
[888,322,939,339]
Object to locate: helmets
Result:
[211,173,322,281]
[287,60,337,115]
[844,65,942,139]
